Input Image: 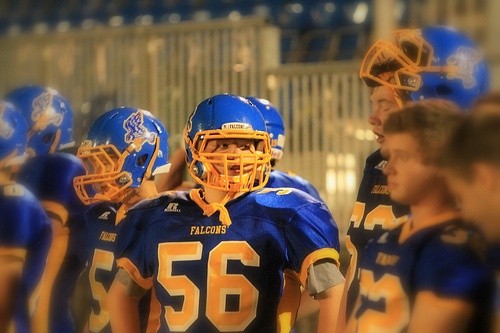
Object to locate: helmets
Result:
[3,86,75,153]
[0,102,28,168]
[243,96,286,162]
[185,95,272,180]
[84,107,171,187]
[358,25,490,109]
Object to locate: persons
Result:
[0,83,345,333]
[425,103,500,332]
[349,96,488,333]
[343,25,489,325]
